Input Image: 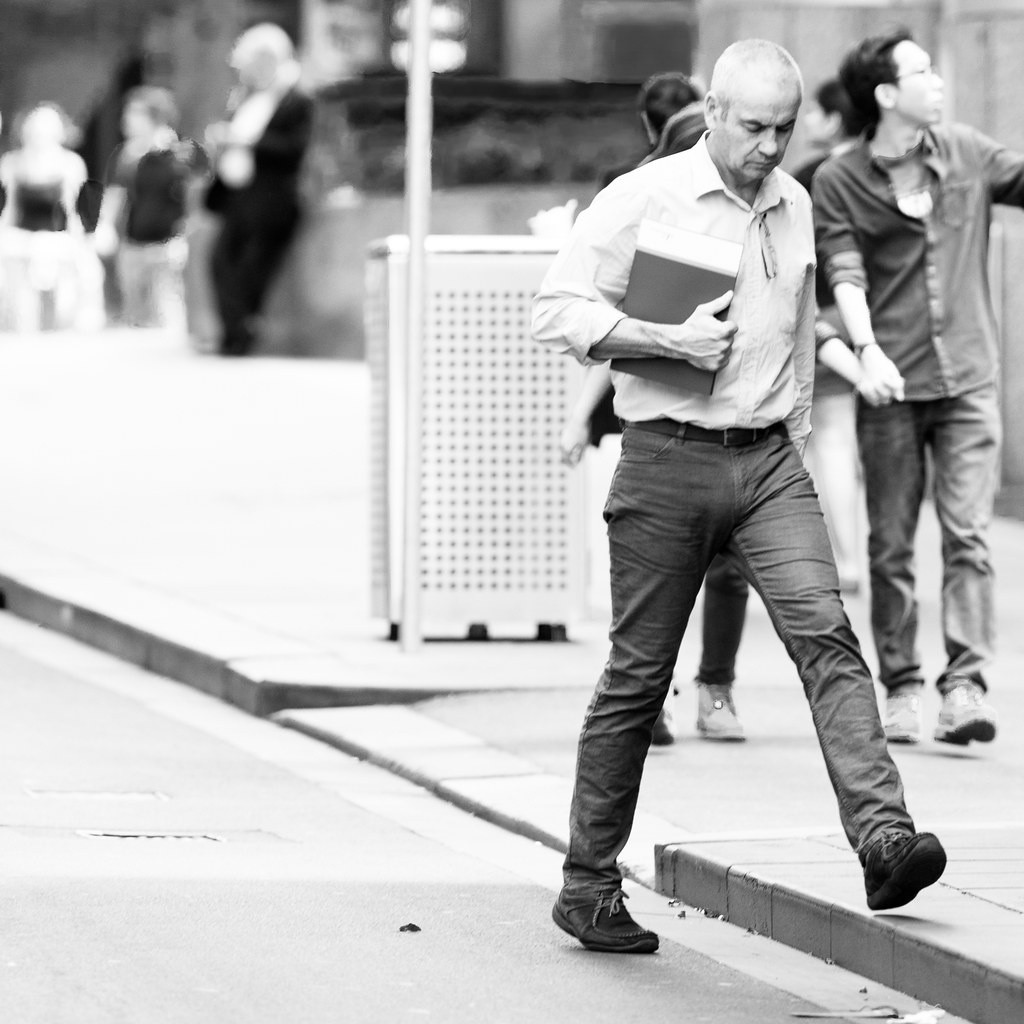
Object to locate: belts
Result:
[628,418,790,446]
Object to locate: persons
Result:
[531,34,948,956]
[209,21,316,354]
[85,86,206,334]
[601,72,704,188]
[788,76,875,598]
[559,96,890,744]
[811,30,1024,746]
[0,101,90,332]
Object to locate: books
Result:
[611,215,744,397]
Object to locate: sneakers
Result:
[694,679,748,739]
[551,885,659,952]
[652,707,673,743]
[934,678,996,743]
[882,682,921,742]
[863,830,947,910]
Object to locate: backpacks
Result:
[128,152,191,245]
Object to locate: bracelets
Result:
[857,344,869,359]
